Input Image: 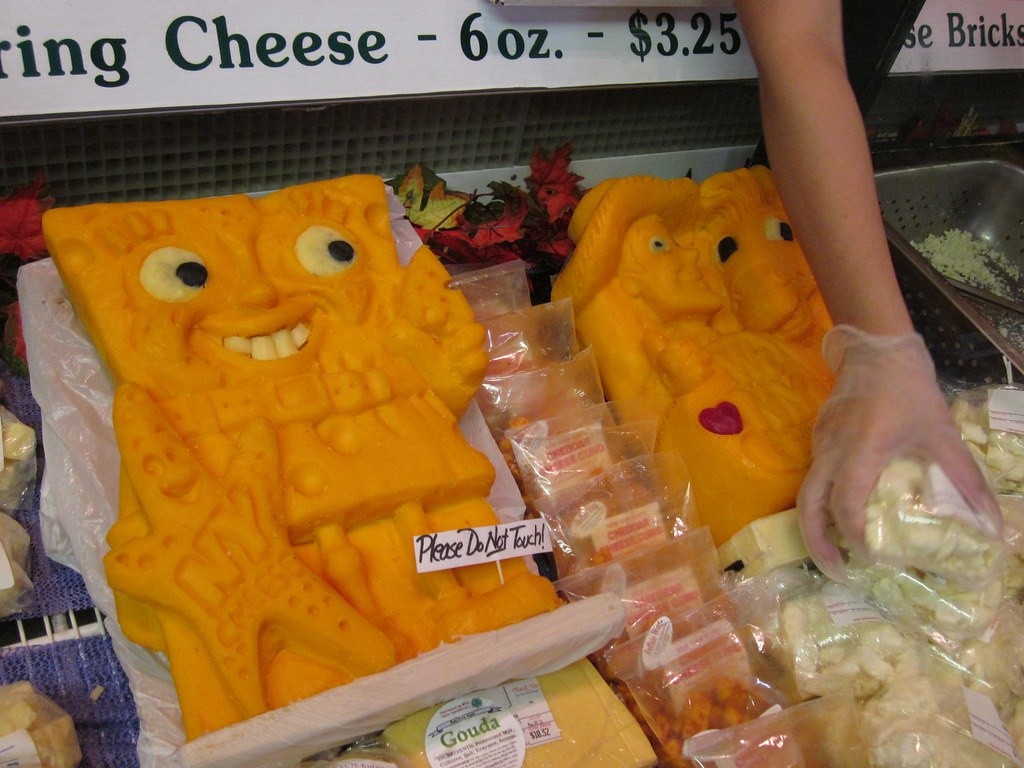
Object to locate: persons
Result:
[740,0,1005,571]
